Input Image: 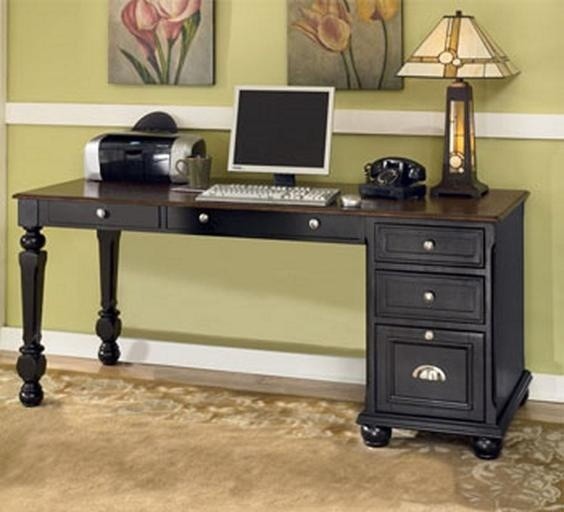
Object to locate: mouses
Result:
[341,193,362,208]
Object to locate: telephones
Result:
[359,156,426,201]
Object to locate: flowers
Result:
[291,0,398,89]
[117,0,201,83]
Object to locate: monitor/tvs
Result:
[226,84,337,185]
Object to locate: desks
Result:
[12,176,532,458]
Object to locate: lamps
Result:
[395,9,521,197]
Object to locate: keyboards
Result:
[194,182,341,207]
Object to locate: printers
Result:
[83,112,207,184]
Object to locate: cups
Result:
[175,156,212,190]
